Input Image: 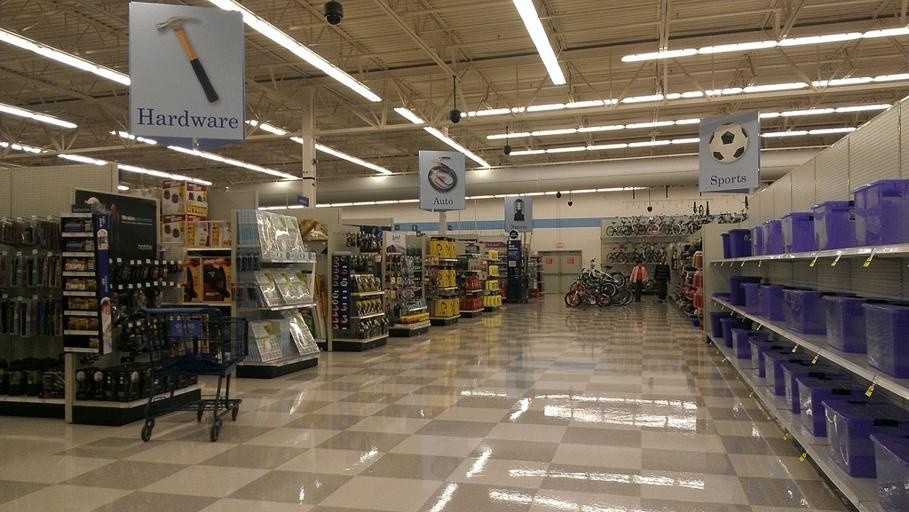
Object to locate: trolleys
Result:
[110,298,251,443]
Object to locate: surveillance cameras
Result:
[568,201,573,206]
[648,206,652,211]
[557,193,561,198]
[450,111,461,123]
[324,2,343,25]
[504,146,511,154]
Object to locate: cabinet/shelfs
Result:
[330,218,459,352]
[502,235,544,304]
[673,215,703,332]
[234,211,329,379]
[460,233,502,318]
[602,215,672,296]
[1,212,233,423]
[704,95,908,512]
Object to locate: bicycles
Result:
[563,203,745,308]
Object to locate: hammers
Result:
[154,14,220,103]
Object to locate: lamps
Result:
[394,107,490,167]
[1,29,130,86]
[323,1,344,25]
[245,120,392,174]
[450,75,461,123]
[513,0,565,86]
[2,142,213,185]
[460,74,908,156]
[620,27,909,63]
[1,103,78,128]
[210,0,383,102]
[109,130,299,180]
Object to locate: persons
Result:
[654,255,672,302]
[629,259,650,304]
